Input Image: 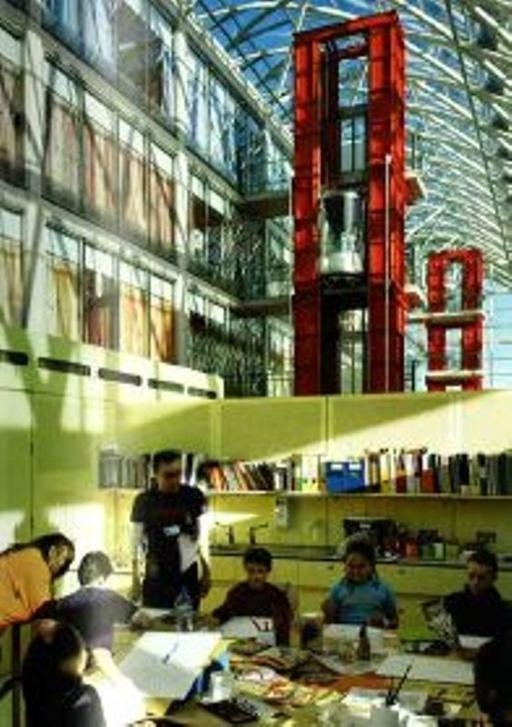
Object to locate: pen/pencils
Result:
[253,619,269,632]
[386,661,414,705]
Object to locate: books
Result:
[102,448,301,493]
[314,446,512,495]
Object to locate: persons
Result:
[20,618,108,727]
[474,627,512,727]
[1,533,76,628]
[124,449,212,617]
[439,551,511,636]
[203,547,291,648]
[321,536,397,628]
[54,549,138,649]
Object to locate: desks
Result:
[164,644,483,726]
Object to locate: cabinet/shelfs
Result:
[299,562,345,612]
[377,564,467,621]
[198,554,238,613]
